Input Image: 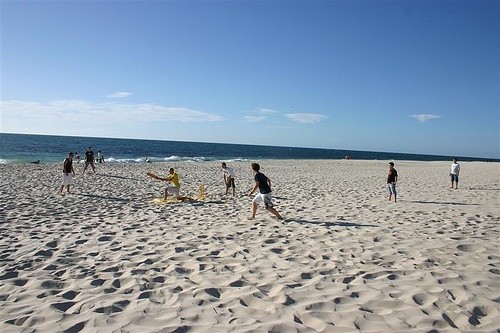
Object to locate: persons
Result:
[60,152,75,196]
[450,158,460,189]
[161,167,194,202]
[386,162,398,202]
[75,147,106,174]
[247,163,283,220]
[221,163,236,196]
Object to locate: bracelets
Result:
[250,191,253,194]
